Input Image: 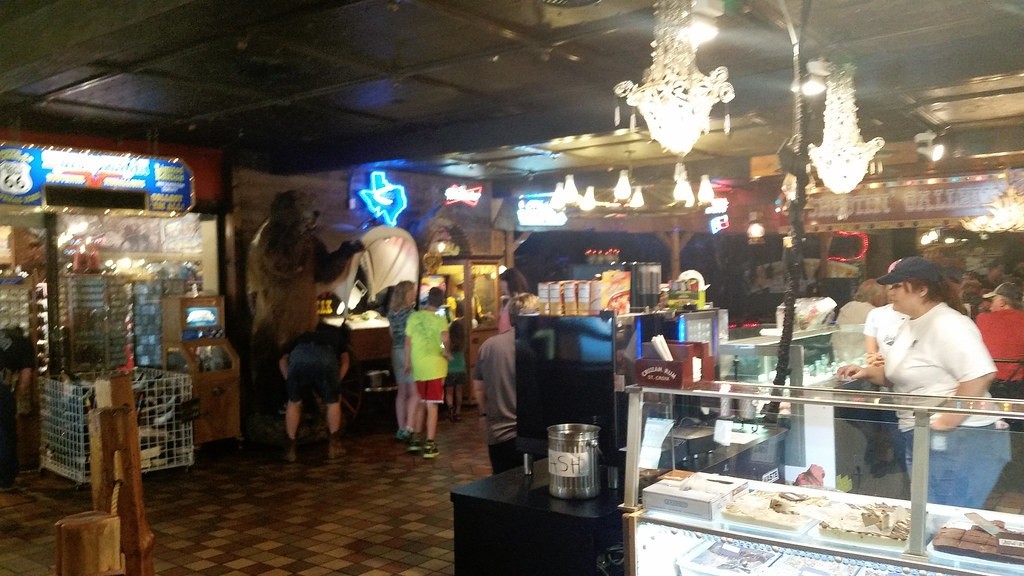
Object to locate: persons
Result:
[974,281,1024,399]
[473,327,523,474]
[280,331,350,462]
[404,287,451,458]
[863,259,908,392]
[445,320,468,422]
[830,279,887,364]
[0,325,35,492]
[983,259,1006,290]
[387,281,417,442]
[836,257,1011,508]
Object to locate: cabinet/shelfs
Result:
[450,457,627,576]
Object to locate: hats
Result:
[876,257,938,287]
[981,282,1021,300]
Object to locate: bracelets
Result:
[479,413,487,416]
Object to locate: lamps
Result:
[614,1,735,157]
[808,65,884,196]
[915,129,946,162]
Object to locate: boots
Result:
[324,430,346,459]
[278,434,296,463]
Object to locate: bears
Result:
[245,187,365,356]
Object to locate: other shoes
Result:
[398,428,412,441]
[448,405,463,422]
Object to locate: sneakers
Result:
[406,435,422,452]
[424,443,439,459]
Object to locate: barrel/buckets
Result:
[548,423,605,501]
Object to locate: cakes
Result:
[818,502,912,548]
[722,489,831,530]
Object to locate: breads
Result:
[932,519,1024,566]
